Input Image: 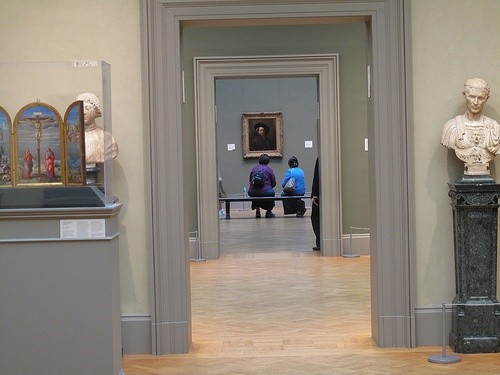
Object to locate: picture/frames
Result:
[241,112,283,160]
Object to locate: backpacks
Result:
[253,168,265,189]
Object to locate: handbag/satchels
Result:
[283,169,295,193]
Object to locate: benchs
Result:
[218,196,311,219]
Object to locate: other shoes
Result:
[313,247,320,251]
[256,211,261,218]
[265,212,275,218]
[301,201,306,216]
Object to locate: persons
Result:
[248,154,276,218]
[281,155,307,218]
[440,78,500,164]
[74,93,119,169]
[218,178,227,215]
[310,157,320,251]
